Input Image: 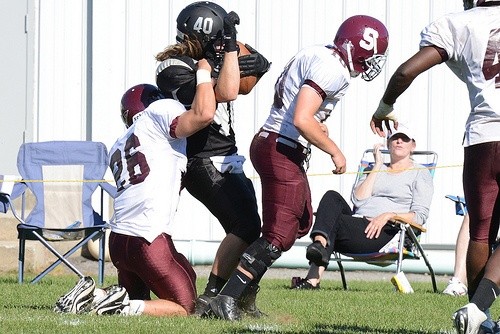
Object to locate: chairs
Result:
[312,149,444,292]
[0,142,118,286]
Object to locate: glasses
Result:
[391,134,415,143]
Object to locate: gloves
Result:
[223,11,240,52]
[238,44,270,78]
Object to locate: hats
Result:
[387,124,416,141]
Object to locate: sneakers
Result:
[292,278,320,290]
[451,302,487,334]
[306,241,329,266]
[480,309,500,334]
[236,286,267,319]
[77,284,131,317]
[53,276,95,315]
[210,294,242,322]
[195,294,218,320]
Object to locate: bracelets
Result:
[196,69,211,86]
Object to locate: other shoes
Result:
[442,276,468,297]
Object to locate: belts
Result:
[258,132,309,154]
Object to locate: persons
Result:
[290,125,433,289]
[209,15,389,320]
[370,0,500,334]
[156,1,270,318]
[443,211,471,295]
[54,58,216,318]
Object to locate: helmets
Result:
[176,1,228,53]
[334,15,389,81]
[120,84,165,129]
[463,0,500,11]
